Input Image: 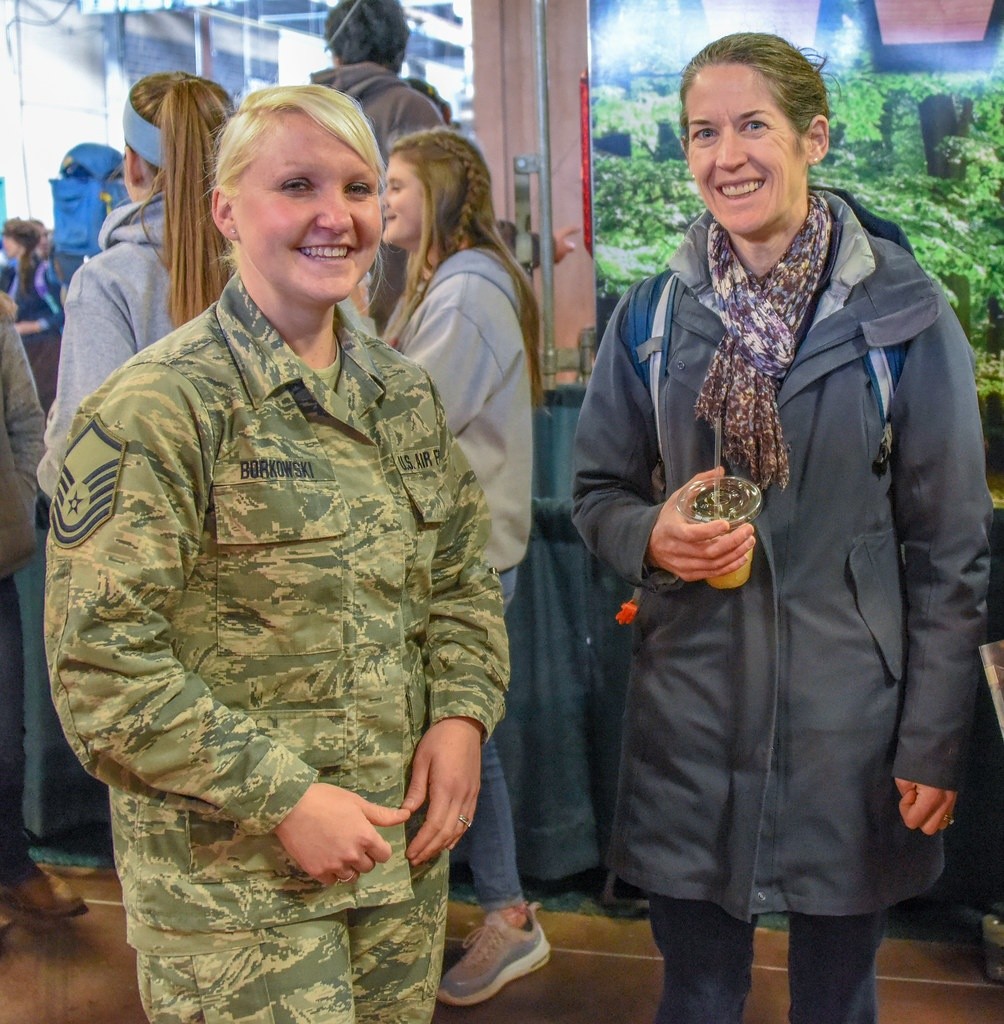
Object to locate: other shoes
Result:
[0,866,90,919]
[0,901,16,929]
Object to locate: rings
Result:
[338,870,355,882]
[943,814,954,824]
[458,815,471,827]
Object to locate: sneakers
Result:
[439,901,550,1007]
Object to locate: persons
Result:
[45,84,511,1024]
[35,72,235,529]
[0,216,65,338]
[0,287,83,932]
[570,32,995,1024]
[380,127,550,1007]
[308,0,448,336]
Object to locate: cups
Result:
[676,475,763,589]
